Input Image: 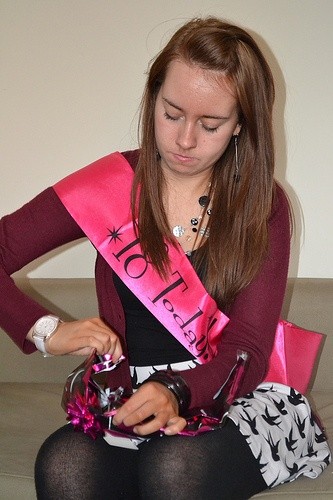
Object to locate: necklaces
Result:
[173,183,213,256]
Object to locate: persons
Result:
[1,17,333,500]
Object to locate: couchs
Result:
[0,279,333,500]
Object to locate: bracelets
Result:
[143,369,193,414]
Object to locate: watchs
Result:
[31,314,63,358]
